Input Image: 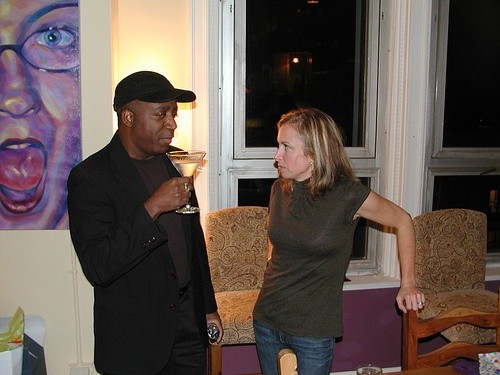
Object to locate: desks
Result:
[0,312,47,375]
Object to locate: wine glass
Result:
[165,150,206,214]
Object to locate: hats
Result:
[114,71,197,111]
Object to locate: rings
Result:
[421,301,425,304]
[417,301,423,305]
[184,182,192,192]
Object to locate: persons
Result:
[64,70,225,375]
[250,105,429,375]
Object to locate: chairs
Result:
[400,287,500,371]
[204,206,269,375]
[411,208,500,346]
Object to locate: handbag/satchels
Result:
[0,345,23,375]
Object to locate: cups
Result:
[355,363,382,375]
